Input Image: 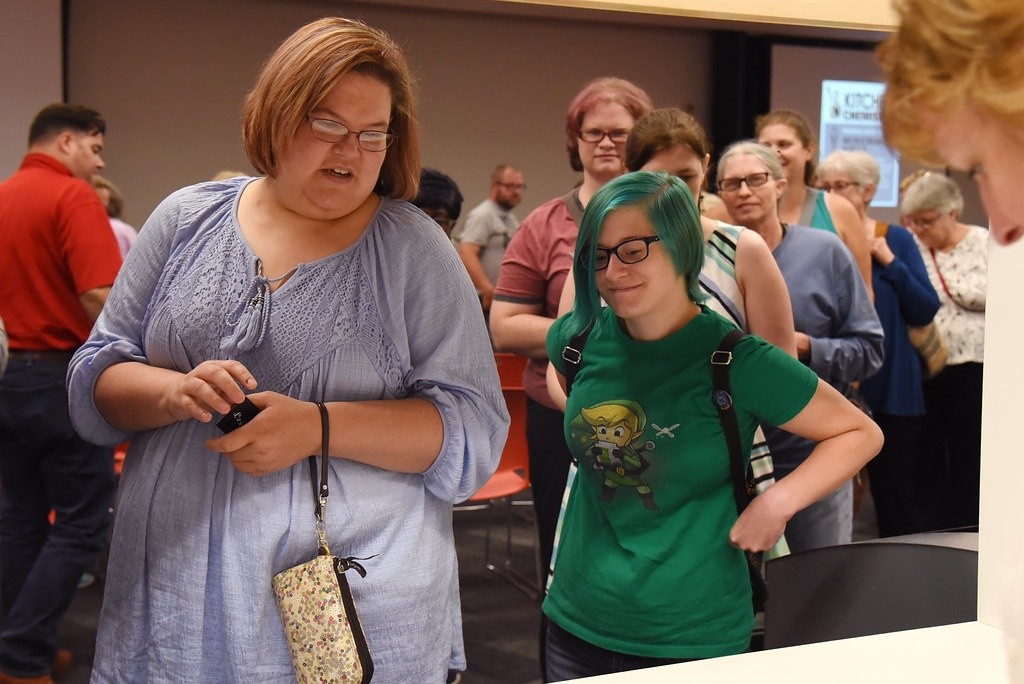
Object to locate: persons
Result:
[546,106,790,605]
[709,108,990,556]
[458,162,525,324]
[407,167,464,240]
[871,0,1023,249]
[65,18,513,684]
[487,75,652,683]
[0,100,139,684]
[547,169,884,684]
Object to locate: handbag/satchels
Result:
[272,401,374,684]
[910,320,948,377]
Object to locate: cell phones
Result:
[216,398,260,434]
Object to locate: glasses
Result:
[581,235,660,271]
[576,127,629,143]
[719,173,771,192]
[496,182,526,189]
[917,214,941,226]
[305,115,398,152]
[822,180,860,193]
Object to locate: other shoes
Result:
[750,612,765,636]
[51,649,74,676]
[1,673,52,684]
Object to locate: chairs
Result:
[453,353,541,603]
